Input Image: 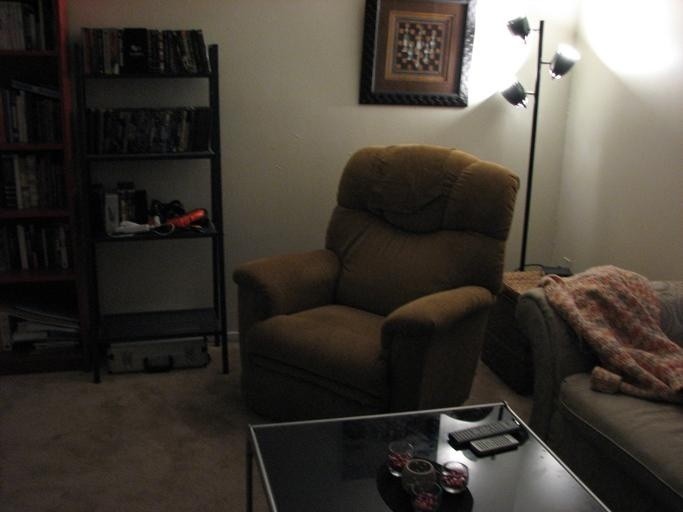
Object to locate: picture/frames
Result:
[337,412,443,485]
[357,0,476,108]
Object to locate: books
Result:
[0,0,82,357]
[64,22,213,157]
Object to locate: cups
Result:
[439,461,469,496]
[404,481,443,510]
[401,458,437,496]
[386,441,416,477]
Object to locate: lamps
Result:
[501,14,581,271]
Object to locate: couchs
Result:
[514,263,682,512]
[230,144,522,426]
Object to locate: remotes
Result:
[470,434,519,456]
[448,419,520,449]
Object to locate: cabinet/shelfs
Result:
[0,1,100,376]
[75,43,229,383]
[477,263,576,398]
